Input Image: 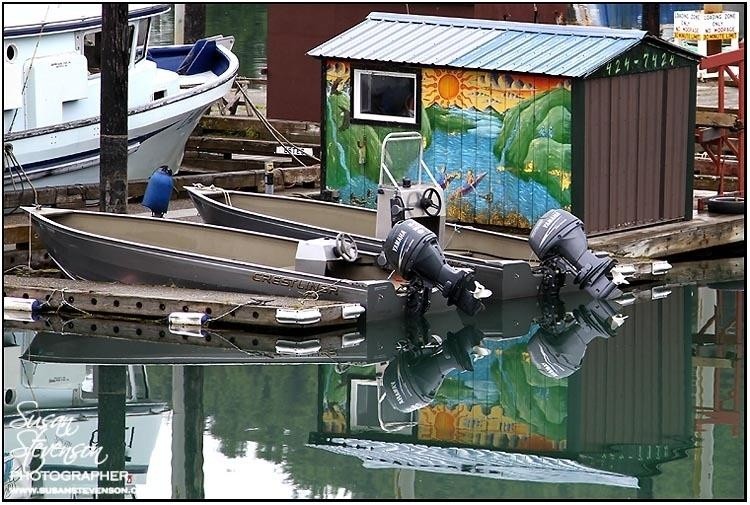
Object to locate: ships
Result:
[2,3,240,191]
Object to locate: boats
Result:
[19,328,492,412]
[437,171,458,188]
[182,132,634,303]
[20,202,492,325]
[451,171,489,194]
